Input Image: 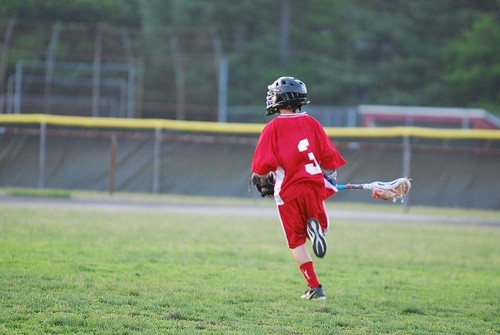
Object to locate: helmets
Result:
[266,76,307,115]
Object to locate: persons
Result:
[249,76,348,300]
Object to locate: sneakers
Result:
[300,284,326,300]
[306,218,327,258]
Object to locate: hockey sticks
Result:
[261,177,410,201]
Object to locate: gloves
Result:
[321,169,337,187]
[252,172,275,199]
